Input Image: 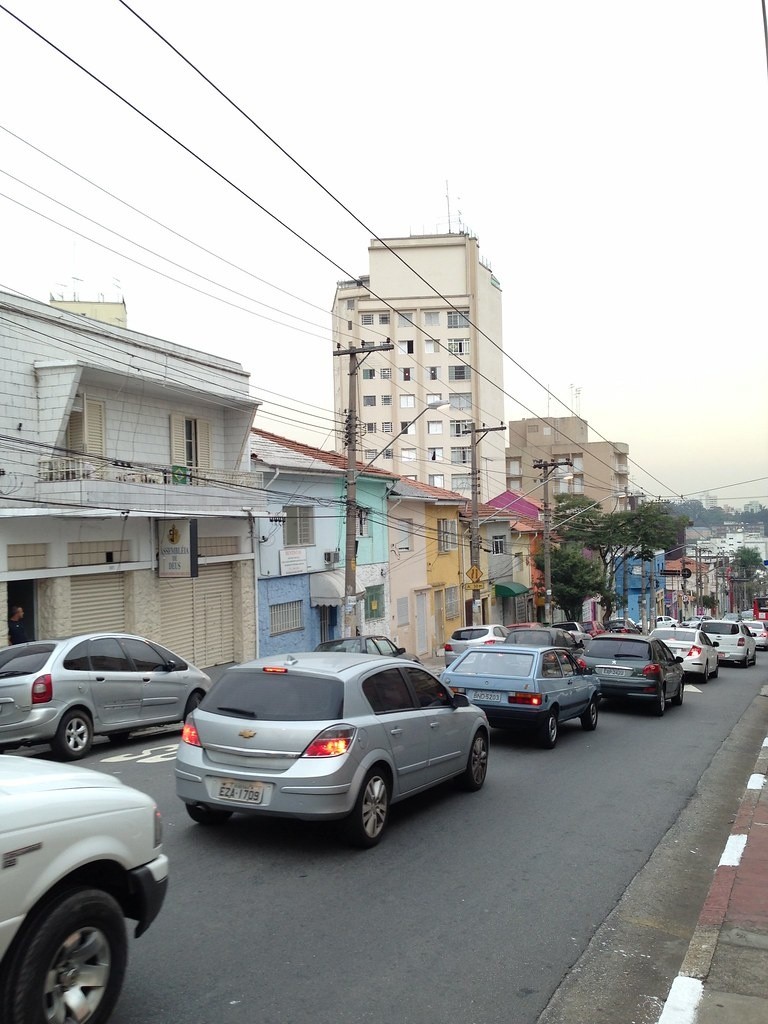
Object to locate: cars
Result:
[506,621,548,630]
[549,618,607,644]
[649,627,719,684]
[720,609,754,621]
[445,624,512,667]
[603,619,642,634]
[636,615,680,632]
[505,629,585,657]
[315,635,422,665]
[174,652,491,849]
[575,632,686,716]
[681,615,716,630]
[440,645,602,749]
[742,620,768,651]
[0,632,212,759]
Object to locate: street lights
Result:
[650,544,696,631]
[344,399,452,639]
[471,473,574,626]
[544,491,627,627]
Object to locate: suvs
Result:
[0,755,168,1024]
[699,620,757,668]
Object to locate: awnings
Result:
[310,569,366,608]
[495,581,528,597]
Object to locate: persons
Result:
[8,606,27,646]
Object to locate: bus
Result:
[753,596,768,622]
